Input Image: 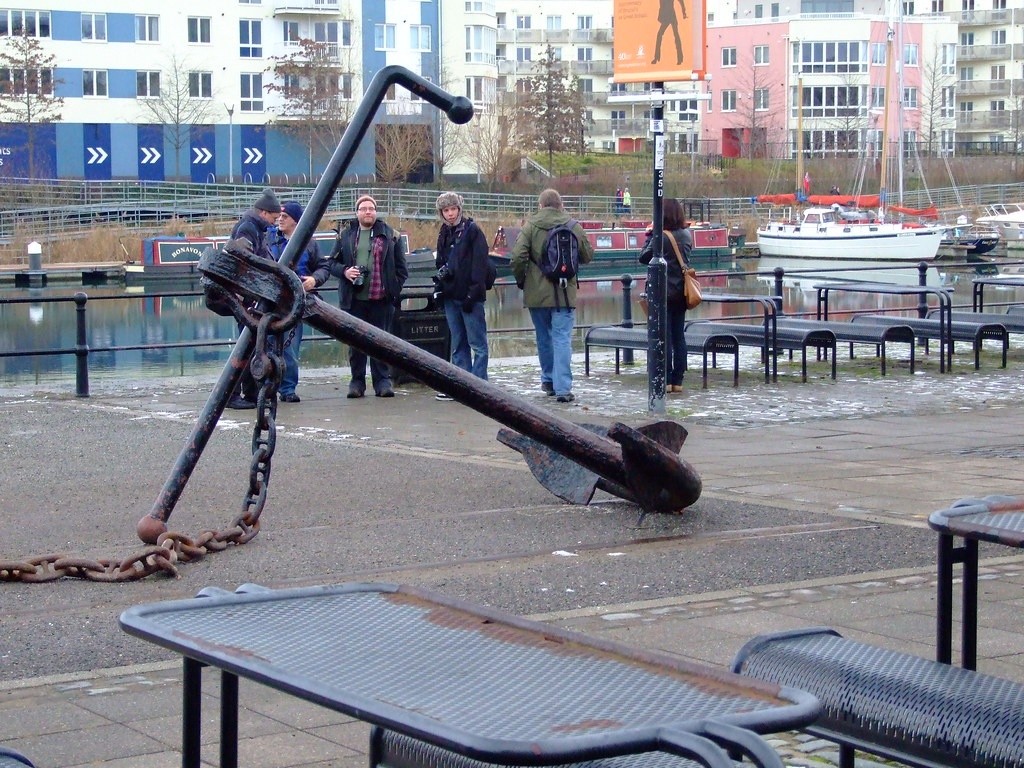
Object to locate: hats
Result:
[436,192,464,227]
[355,195,377,212]
[281,201,303,223]
[254,188,281,213]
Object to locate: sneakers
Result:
[541,382,555,396]
[557,393,575,402]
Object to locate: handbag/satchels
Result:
[200,276,236,316]
[682,268,702,310]
[431,258,496,307]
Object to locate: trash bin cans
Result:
[390,289,451,388]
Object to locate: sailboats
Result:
[752,0,1001,261]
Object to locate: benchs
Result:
[585,303,1024,388]
[726,626,1024,768]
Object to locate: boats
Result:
[140,213,764,280]
[974,201,1024,239]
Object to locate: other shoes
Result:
[224,396,256,409]
[347,387,364,398]
[673,384,682,392]
[244,395,258,404]
[435,393,455,401]
[375,386,394,397]
[279,393,300,403]
[667,384,673,393]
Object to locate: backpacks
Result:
[528,219,579,313]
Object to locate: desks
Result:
[972,278,1024,350]
[640,292,782,384]
[814,282,955,373]
[120,581,822,768]
[927,495,1024,671]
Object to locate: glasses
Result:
[357,207,377,212]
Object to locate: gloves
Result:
[436,265,450,281]
[463,295,474,314]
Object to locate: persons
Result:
[640,198,692,392]
[226,187,281,409]
[615,187,631,217]
[268,202,331,403]
[510,189,594,402]
[435,193,488,401]
[831,186,839,195]
[329,195,408,398]
